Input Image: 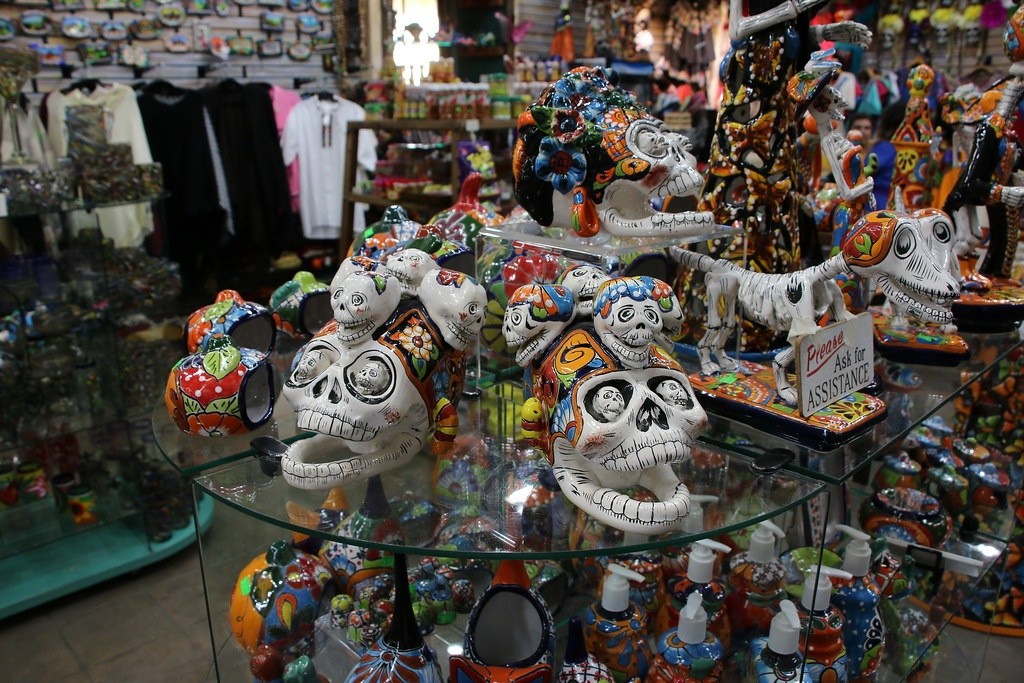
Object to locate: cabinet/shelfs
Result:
[0,0,1024,682]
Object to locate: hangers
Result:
[298,76,339,100]
[142,61,186,98]
